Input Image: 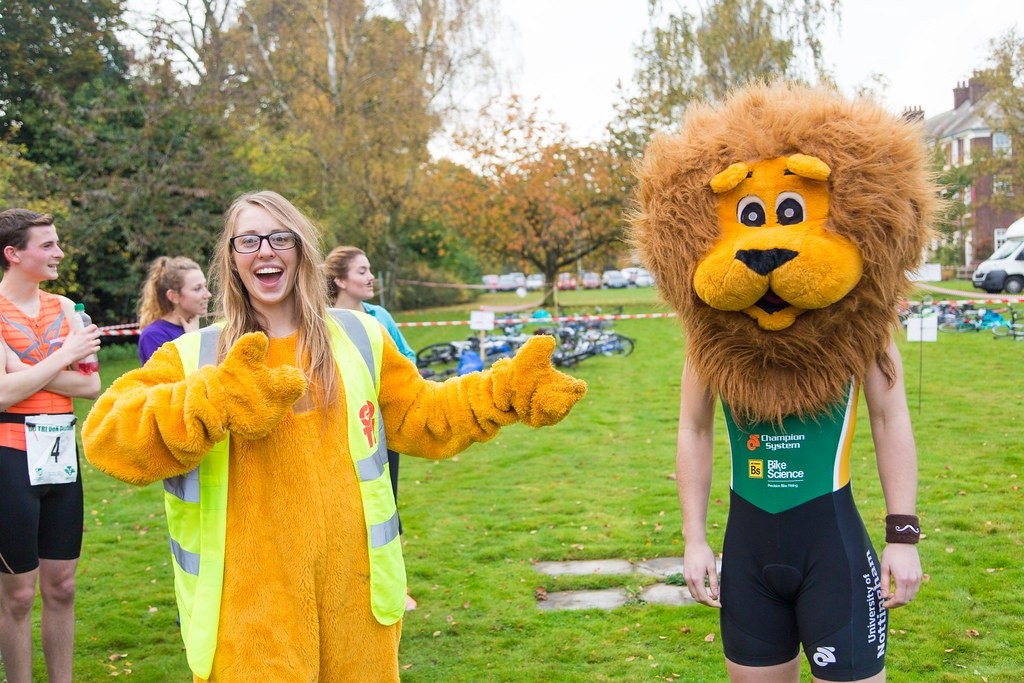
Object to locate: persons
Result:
[317,246,417,612]
[136,256,213,367]
[630,86,947,683]
[1,209,103,683]
[79,191,587,683]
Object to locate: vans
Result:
[972,216,1024,295]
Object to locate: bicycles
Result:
[412,303,635,383]
[896,296,1023,342]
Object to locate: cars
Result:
[480,264,654,292]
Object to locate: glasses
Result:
[230,231,297,254]
